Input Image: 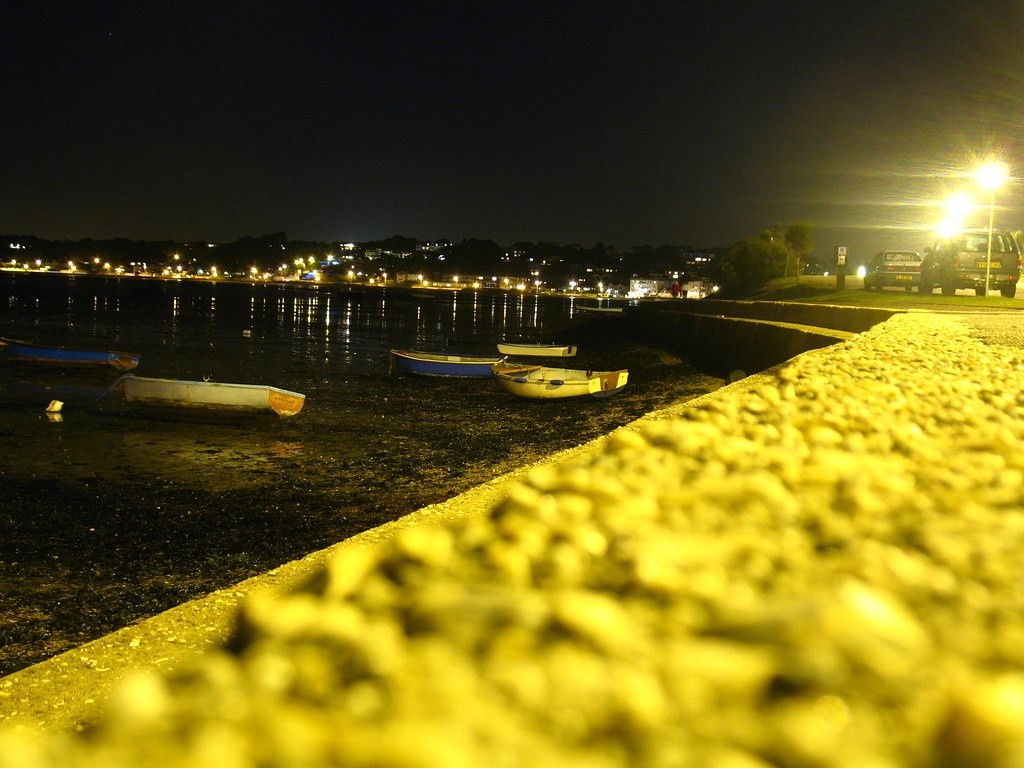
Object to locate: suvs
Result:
[918,225,1022,298]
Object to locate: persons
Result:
[682,282,688,298]
[672,281,679,299]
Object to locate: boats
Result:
[5,338,142,372]
[118,374,307,417]
[497,341,578,357]
[388,348,508,378]
[491,362,630,402]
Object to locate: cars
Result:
[863,250,922,291]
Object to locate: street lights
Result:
[978,160,1008,298]
